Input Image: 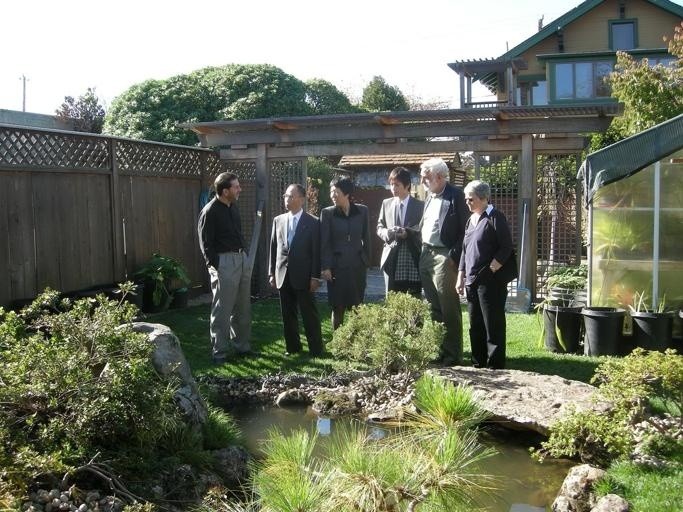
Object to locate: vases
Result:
[169,288,187,308]
[679,310,683,353]
[543,299,585,354]
[102,283,145,316]
[581,306,627,357]
[631,311,673,353]
[74,287,103,311]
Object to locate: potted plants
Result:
[129,249,191,312]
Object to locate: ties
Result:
[287,217,295,248]
[396,203,404,226]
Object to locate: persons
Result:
[372,166,428,303]
[196,171,262,366]
[453,178,520,371]
[414,156,474,369]
[266,183,335,360]
[318,174,373,332]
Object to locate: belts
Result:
[422,243,445,249]
[231,248,243,253]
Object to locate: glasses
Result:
[464,199,472,202]
[282,194,302,199]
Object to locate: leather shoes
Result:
[237,350,261,357]
